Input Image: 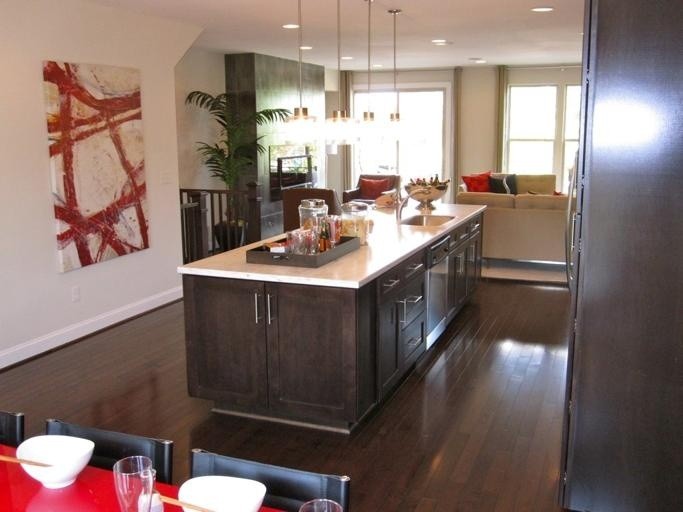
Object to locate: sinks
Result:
[401,214,454,227]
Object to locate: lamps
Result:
[283,0,417,143]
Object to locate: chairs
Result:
[343,174,400,204]
[189,448,351,512]
[282,188,341,233]
[0,409,24,448]
[45,418,174,485]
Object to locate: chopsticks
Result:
[0,455,50,469]
[157,491,213,512]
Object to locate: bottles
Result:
[409,173,450,186]
[137,468,164,512]
[297,197,370,250]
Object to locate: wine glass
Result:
[405,183,448,211]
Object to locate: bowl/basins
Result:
[175,473,269,512]
[15,433,95,490]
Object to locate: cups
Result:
[286,227,321,256]
[111,455,154,512]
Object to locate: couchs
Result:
[457,175,577,269]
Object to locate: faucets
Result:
[395,187,432,220]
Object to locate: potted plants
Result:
[184,91,293,252]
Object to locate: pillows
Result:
[488,173,517,195]
[461,170,492,193]
[359,177,391,200]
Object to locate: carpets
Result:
[481,259,569,285]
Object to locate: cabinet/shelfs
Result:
[183,274,357,428]
[448,212,483,324]
[376,249,426,402]
